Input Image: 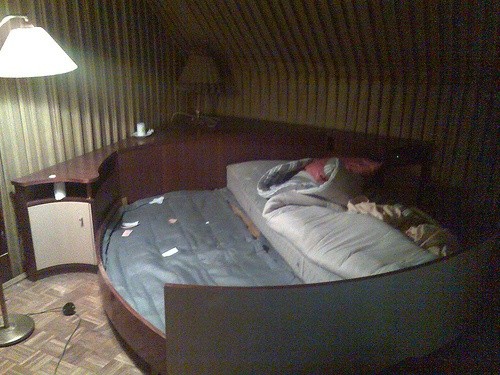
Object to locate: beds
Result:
[12,114,500,375]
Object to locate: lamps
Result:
[0,13,78,348]
[178,54,223,114]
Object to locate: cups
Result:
[137,123,145,136]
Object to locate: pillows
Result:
[305,158,382,184]
